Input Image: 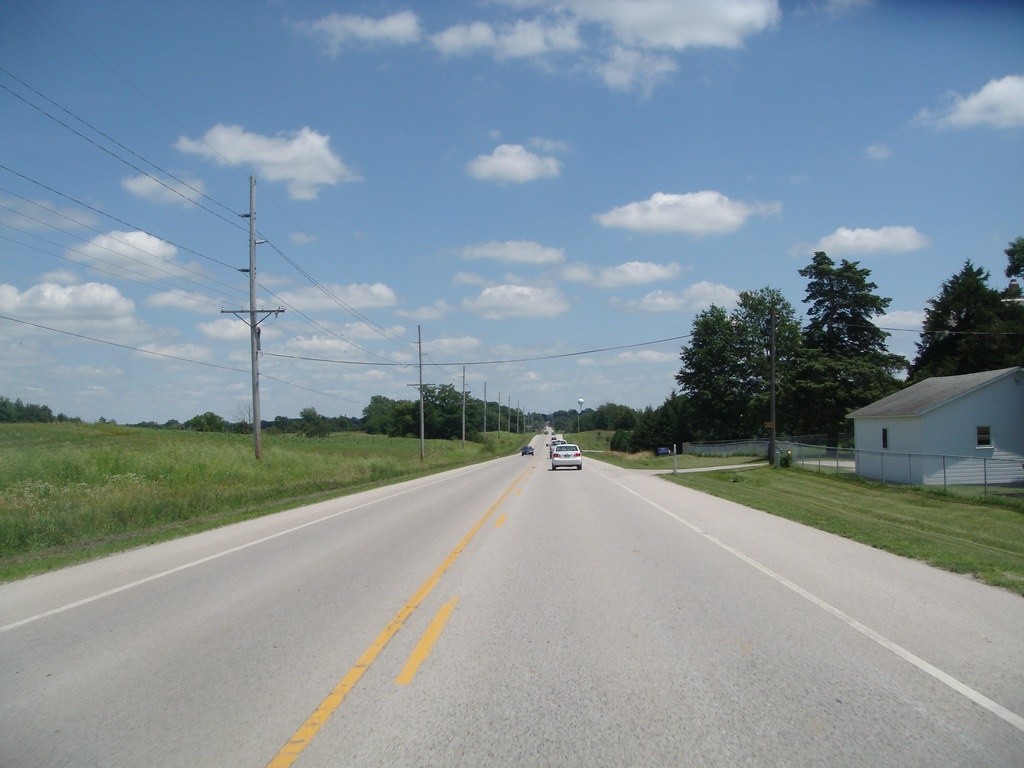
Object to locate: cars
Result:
[550,440,567,459]
[552,443,583,470]
[521,446,534,456]
